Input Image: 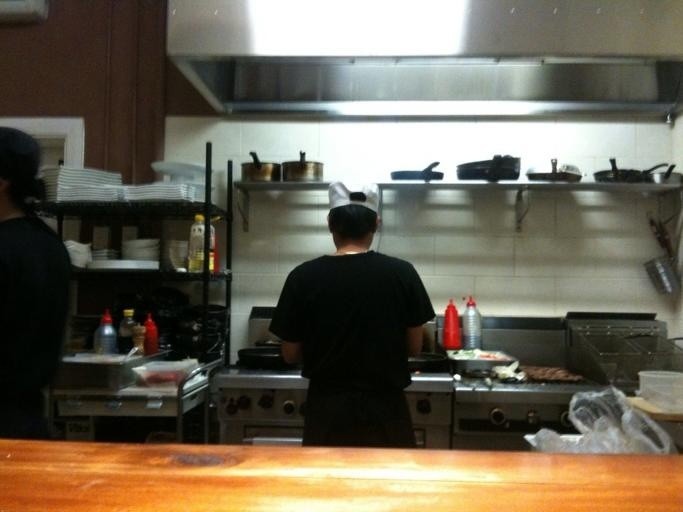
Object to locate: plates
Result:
[91,248,121,260]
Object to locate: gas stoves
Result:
[216,354,456,421]
[455,371,596,428]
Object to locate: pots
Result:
[390,154,683,186]
[240,151,324,184]
[238,346,296,372]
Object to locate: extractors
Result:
[164,0,682,123]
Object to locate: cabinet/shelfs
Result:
[37,141,233,442]
[231,177,683,233]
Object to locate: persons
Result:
[261,179,433,448]
[0,126,74,434]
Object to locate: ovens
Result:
[220,422,453,450]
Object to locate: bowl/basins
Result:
[123,238,158,264]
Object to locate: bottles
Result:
[443,296,481,350]
[188,214,214,273]
[95,309,158,356]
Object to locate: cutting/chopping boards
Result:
[623,395,682,423]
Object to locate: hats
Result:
[0,126,43,182]
[327,179,380,212]
